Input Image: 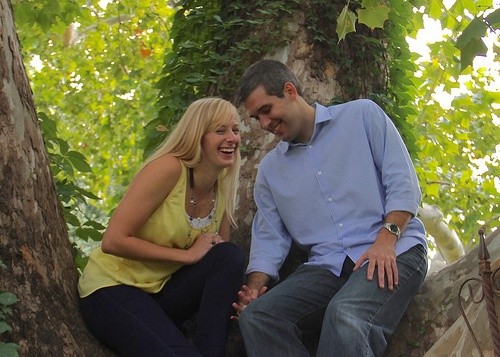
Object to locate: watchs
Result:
[379,223,401,241]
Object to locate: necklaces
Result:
[185,168,217,250]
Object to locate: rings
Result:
[212,237,217,245]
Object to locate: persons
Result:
[77,99,248,357]
[232,59,428,357]
